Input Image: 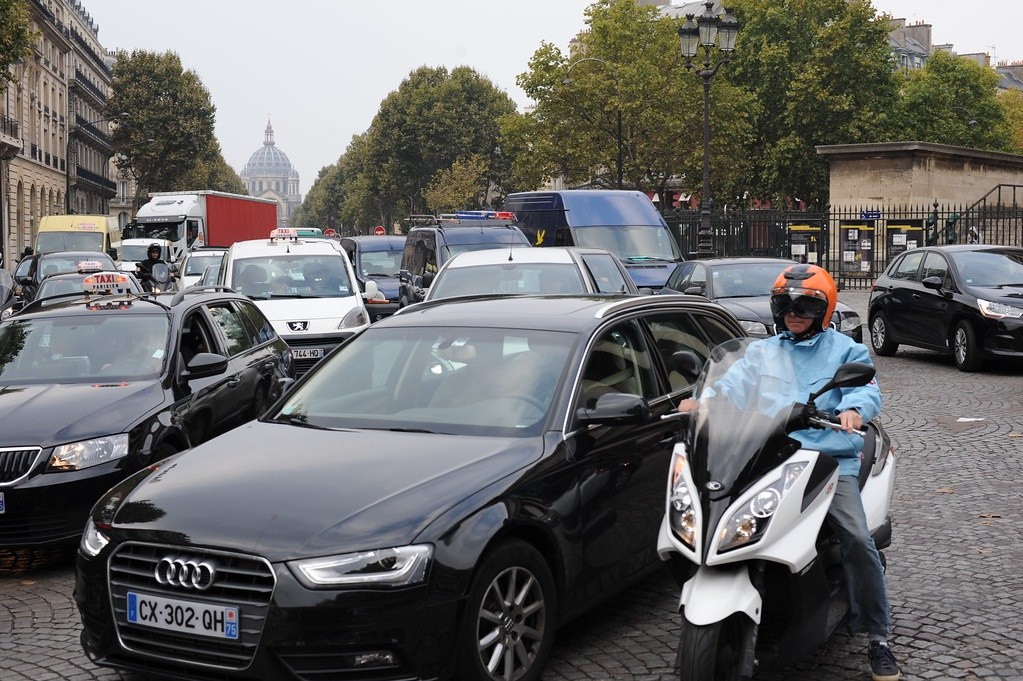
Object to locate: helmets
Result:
[147,243,162,260]
[769,264,838,331]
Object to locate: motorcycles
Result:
[653,357,893,681]
[135,250,184,294]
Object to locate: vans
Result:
[498,186,676,292]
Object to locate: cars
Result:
[424,244,633,296]
[31,271,142,298]
[2,296,300,564]
[175,249,227,292]
[865,241,1023,370]
[399,210,532,306]
[343,231,409,317]
[65,293,757,680]
[217,226,376,389]
[16,214,177,292]
[664,257,865,348]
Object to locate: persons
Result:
[301,263,323,294]
[677,265,900,681]
[135,243,174,292]
[967,226,982,244]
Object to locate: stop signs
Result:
[324,228,337,238]
[374,225,385,235]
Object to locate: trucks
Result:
[129,190,281,249]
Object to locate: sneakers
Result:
[868,640,904,681]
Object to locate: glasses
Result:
[771,294,828,319]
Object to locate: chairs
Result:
[46,264,58,273]
[581,337,638,396]
[304,263,330,287]
[241,267,268,289]
[54,282,73,294]
[429,337,506,406]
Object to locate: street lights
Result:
[678,1,744,252]
